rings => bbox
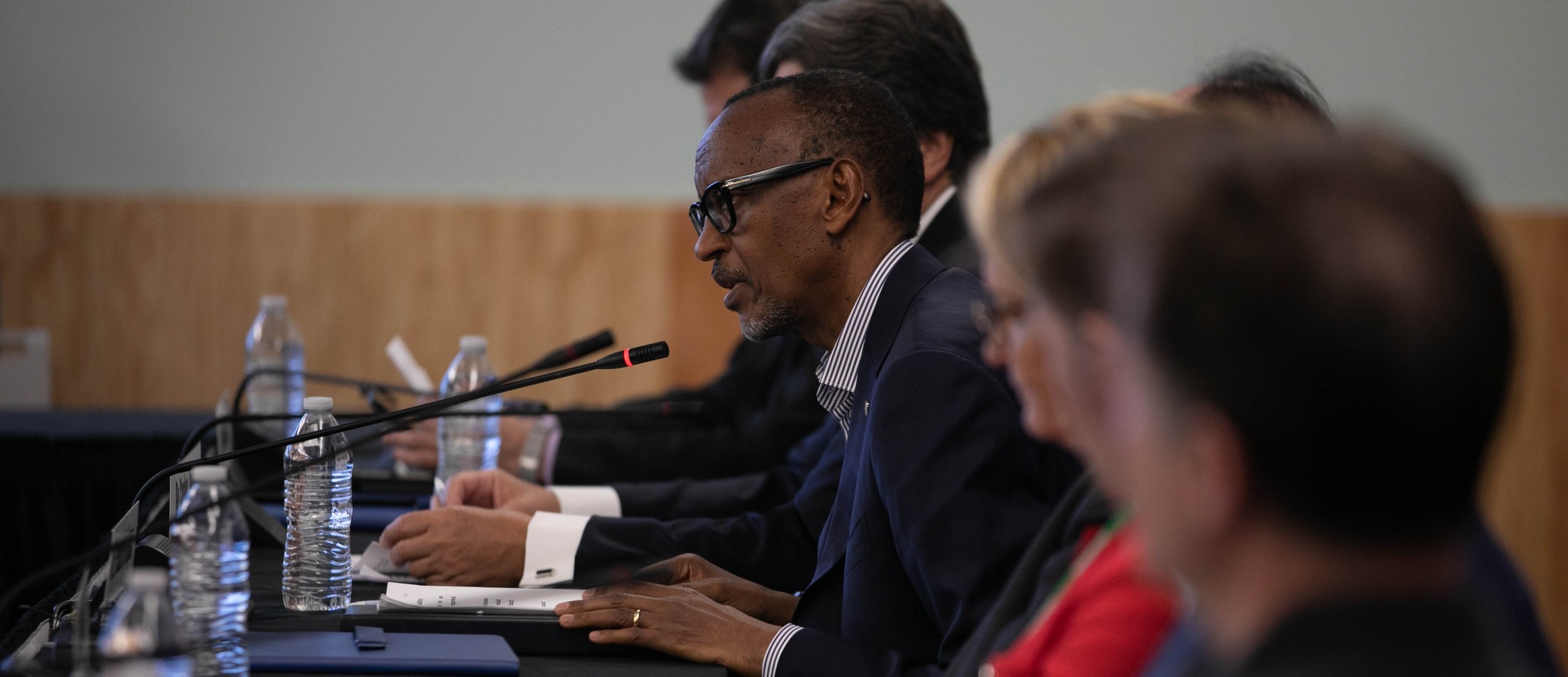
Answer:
[632,608,641,627]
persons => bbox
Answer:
[970,91,1227,677]
[1107,123,1520,677]
[556,64,1039,677]
[367,0,1346,677]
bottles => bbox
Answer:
[98,568,193,677]
[433,336,500,505]
[242,295,304,442]
[281,397,353,611]
[169,466,249,677]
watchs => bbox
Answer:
[516,424,554,487]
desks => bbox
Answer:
[0,405,734,677]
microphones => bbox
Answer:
[132,329,670,531]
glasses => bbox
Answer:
[972,291,1039,349]
[688,158,871,236]
